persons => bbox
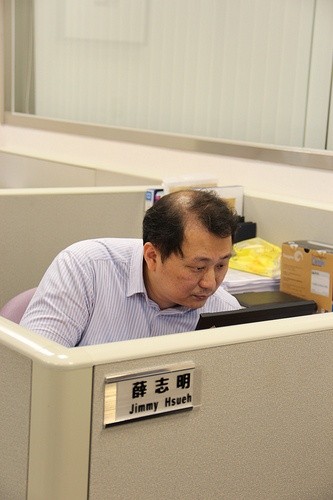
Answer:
[15,191,244,349]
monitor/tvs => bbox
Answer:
[193,301,319,331]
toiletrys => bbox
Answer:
[154,176,177,207]
